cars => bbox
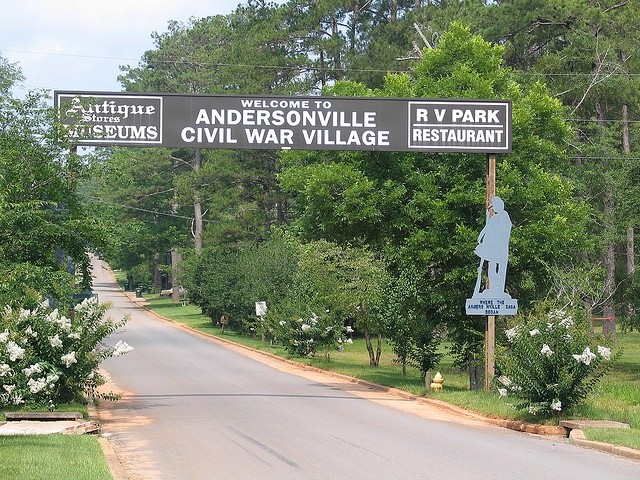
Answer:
[161,286,188,298]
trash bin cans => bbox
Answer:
[136,288,143,297]
[124,282,130,290]
